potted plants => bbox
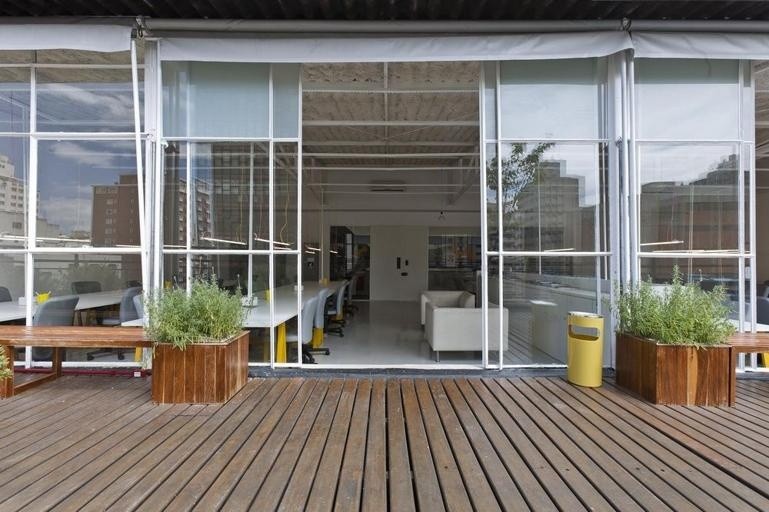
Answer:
[604,264,733,406]
[142,273,253,406]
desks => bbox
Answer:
[233,280,342,361]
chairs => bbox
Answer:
[0,279,140,364]
[418,285,508,361]
[288,274,361,365]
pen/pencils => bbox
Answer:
[35,291,52,295]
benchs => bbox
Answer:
[729,332,769,404]
[1,325,159,397]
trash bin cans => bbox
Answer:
[567,310,604,387]
